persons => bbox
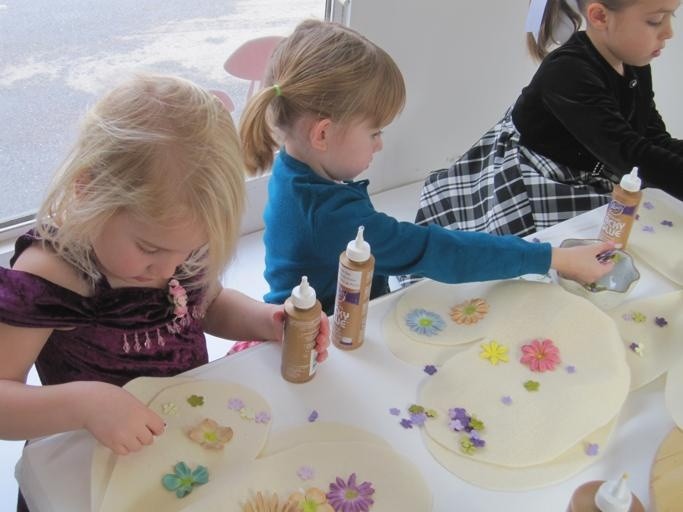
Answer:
[231,18,616,300]
[2,72,334,458]
[401,1,680,236]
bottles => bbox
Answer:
[279,276,322,384]
[598,165,641,250]
[331,226,375,351]
[564,470,646,512]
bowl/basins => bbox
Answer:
[554,236,639,309]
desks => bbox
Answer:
[18,187,680,510]
